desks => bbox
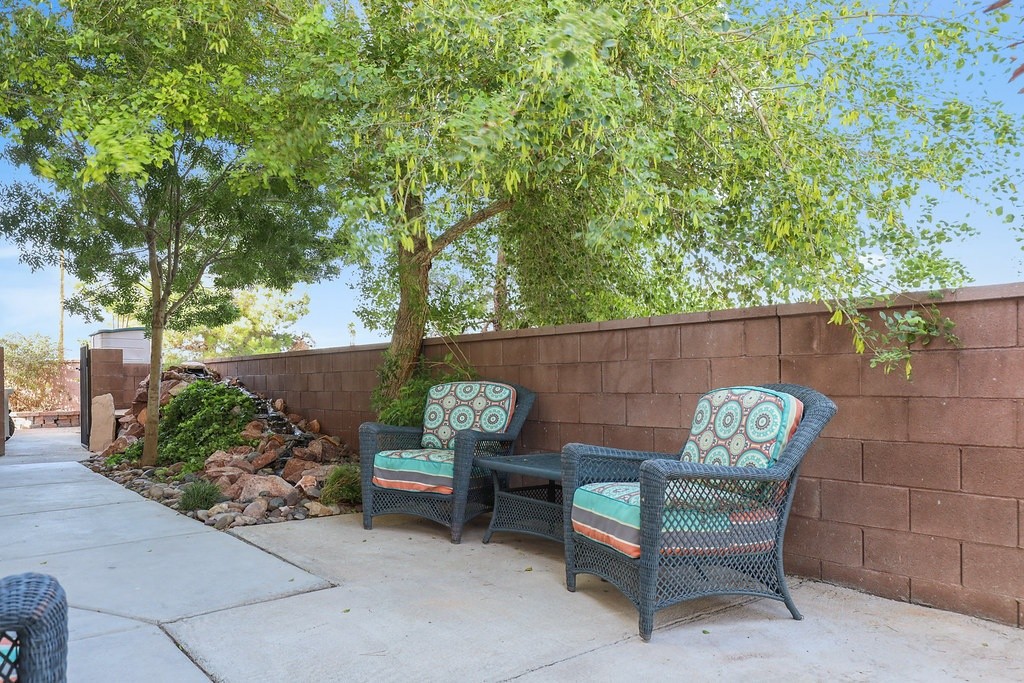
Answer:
[478,453,654,550]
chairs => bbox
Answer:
[563,382,838,641]
[359,379,538,543]
